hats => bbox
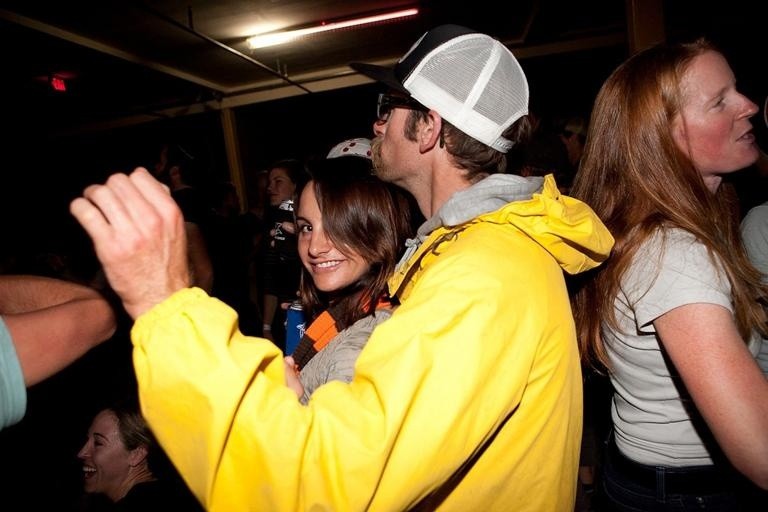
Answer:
[327,137,373,159]
[351,25,529,154]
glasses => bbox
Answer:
[376,93,424,120]
